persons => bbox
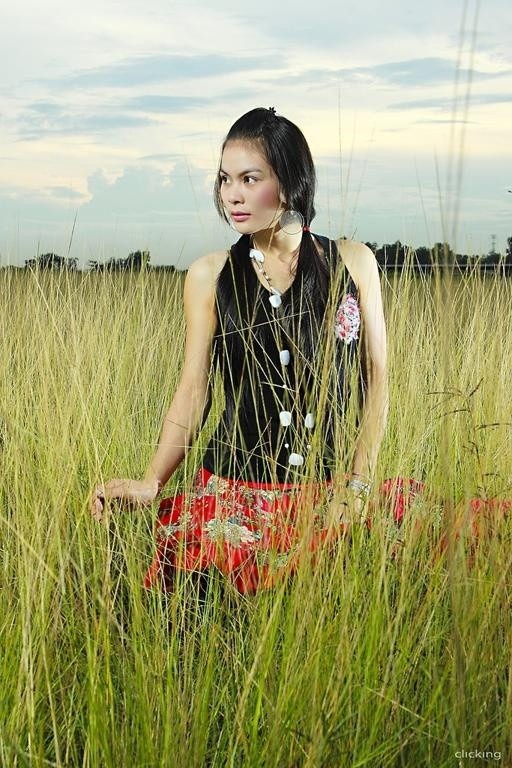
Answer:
[86,103,393,616]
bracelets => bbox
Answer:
[352,471,372,480]
[346,481,371,497]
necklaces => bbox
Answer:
[249,233,319,471]
